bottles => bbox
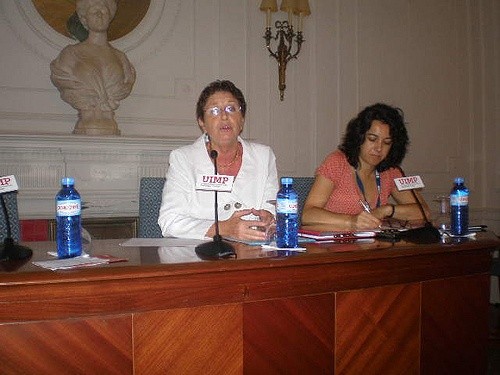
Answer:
[276,177,299,255]
[449,177,469,242]
[55,177,83,259]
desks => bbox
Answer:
[1,235,500,374]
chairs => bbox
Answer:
[138,177,166,237]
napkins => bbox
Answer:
[440,230,476,237]
[261,241,307,252]
[47,250,90,258]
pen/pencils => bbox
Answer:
[359,200,382,228]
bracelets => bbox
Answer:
[386,203,395,218]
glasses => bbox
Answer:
[204,105,241,119]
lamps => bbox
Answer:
[259,0,311,102]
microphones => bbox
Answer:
[194,150,236,257]
[389,162,440,246]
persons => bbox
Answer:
[157,81,280,242]
[301,103,431,230]
[49,0,139,136]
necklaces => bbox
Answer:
[208,139,240,167]
